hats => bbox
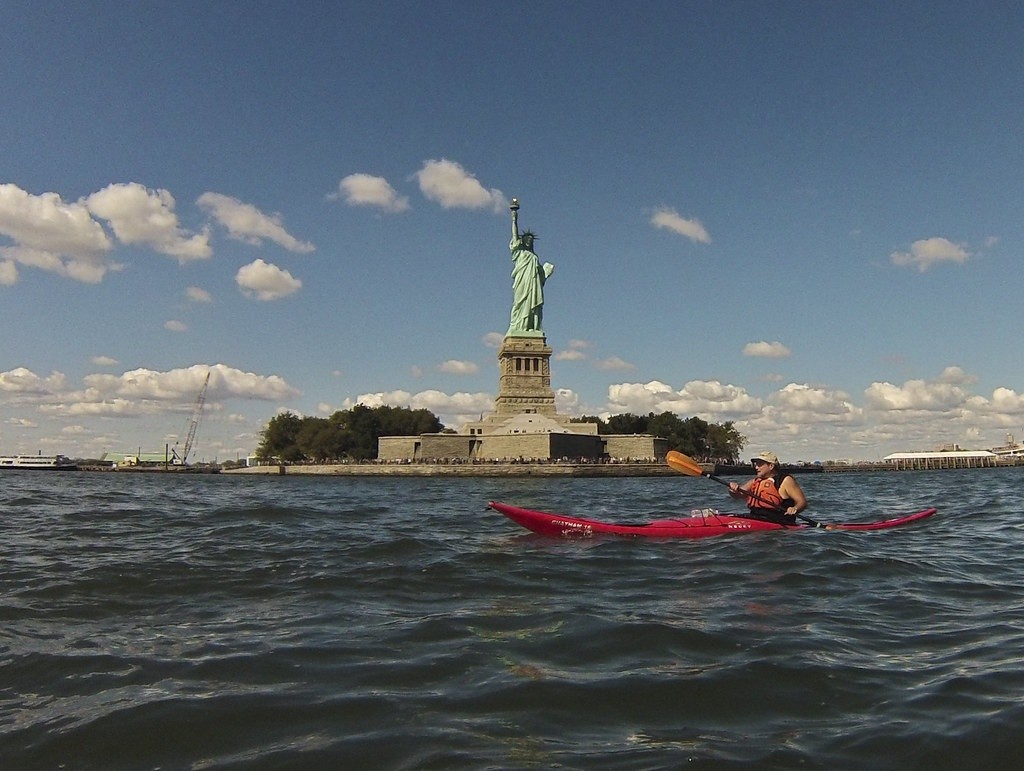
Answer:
[751,452,780,470]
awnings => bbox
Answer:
[883,451,997,461]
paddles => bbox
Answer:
[665,449,832,531]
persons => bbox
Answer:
[506,199,556,336]
[727,451,807,525]
[256,457,739,466]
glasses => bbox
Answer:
[754,460,767,466]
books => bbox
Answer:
[543,262,555,278]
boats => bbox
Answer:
[1,452,80,469]
[485,498,937,536]
